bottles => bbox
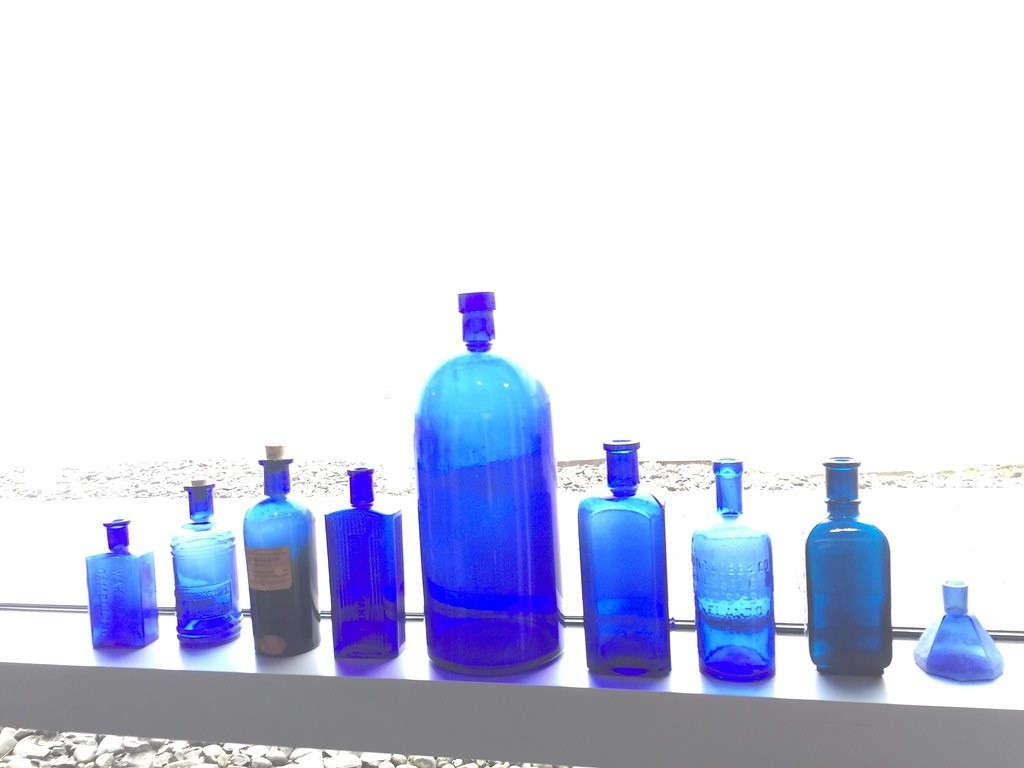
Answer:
[169,479,243,650]
[691,457,775,683]
[243,444,321,658]
[913,579,1004,682]
[324,467,407,659]
[577,440,672,679]
[415,291,565,677]
[85,518,160,649]
[805,454,893,677]
